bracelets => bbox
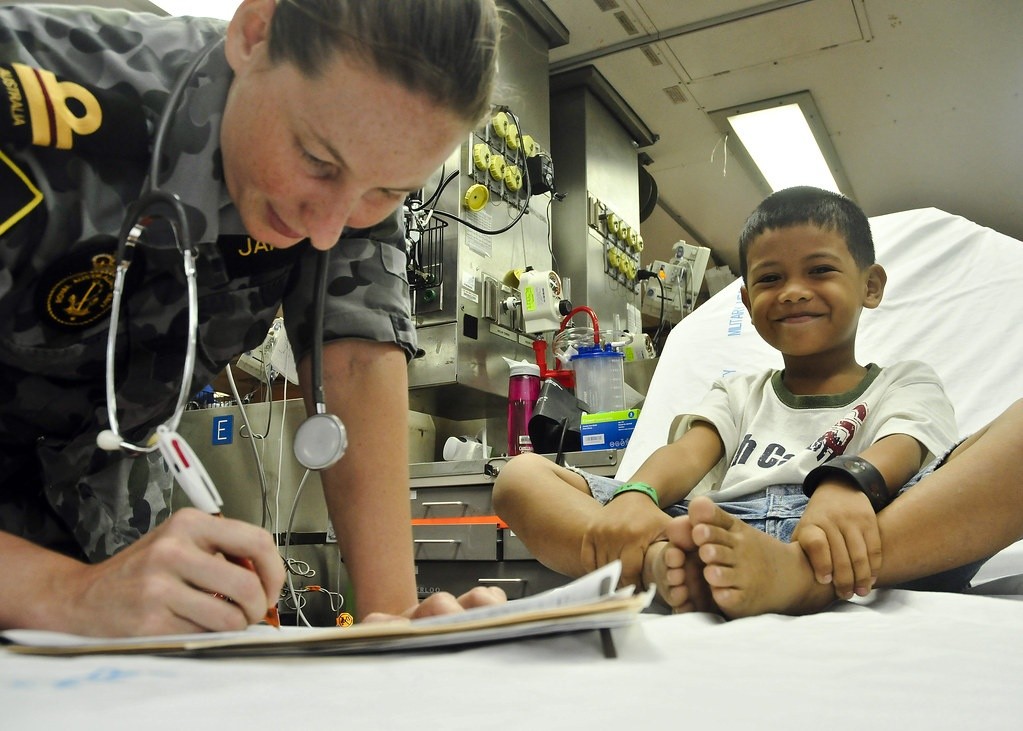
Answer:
[803,455,888,513]
[604,482,659,507]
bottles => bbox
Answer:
[504,357,541,456]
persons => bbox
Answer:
[0,0,505,636]
[492,185,1023,620]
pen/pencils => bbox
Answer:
[158,432,280,631]
